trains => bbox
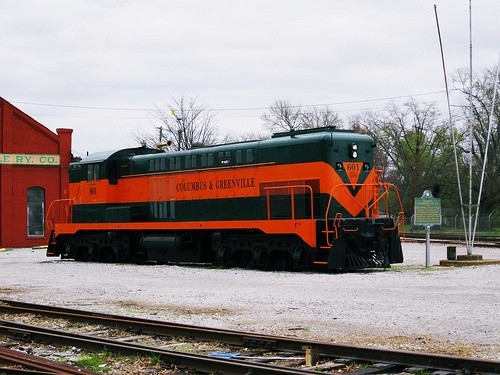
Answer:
[41,122,405,274]
